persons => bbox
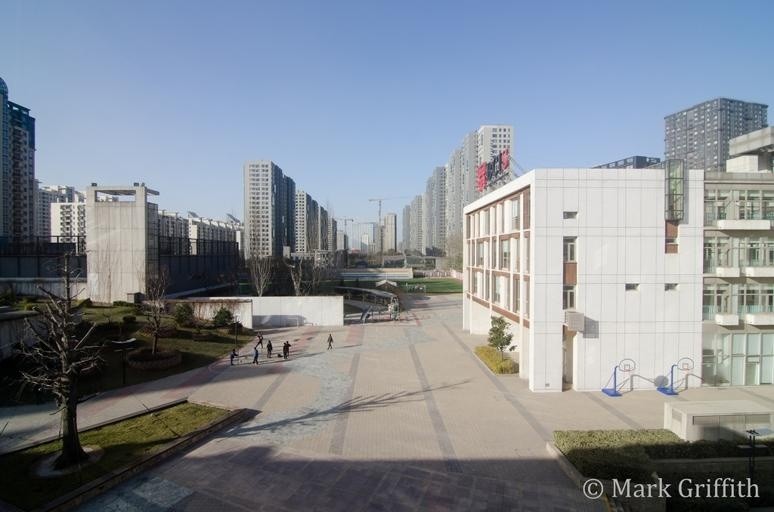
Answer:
[283,342,287,359]
[255,331,263,347]
[266,341,273,358]
[326,333,333,350]
[252,347,258,366]
[285,340,291,356]
[230,347,238,366]
